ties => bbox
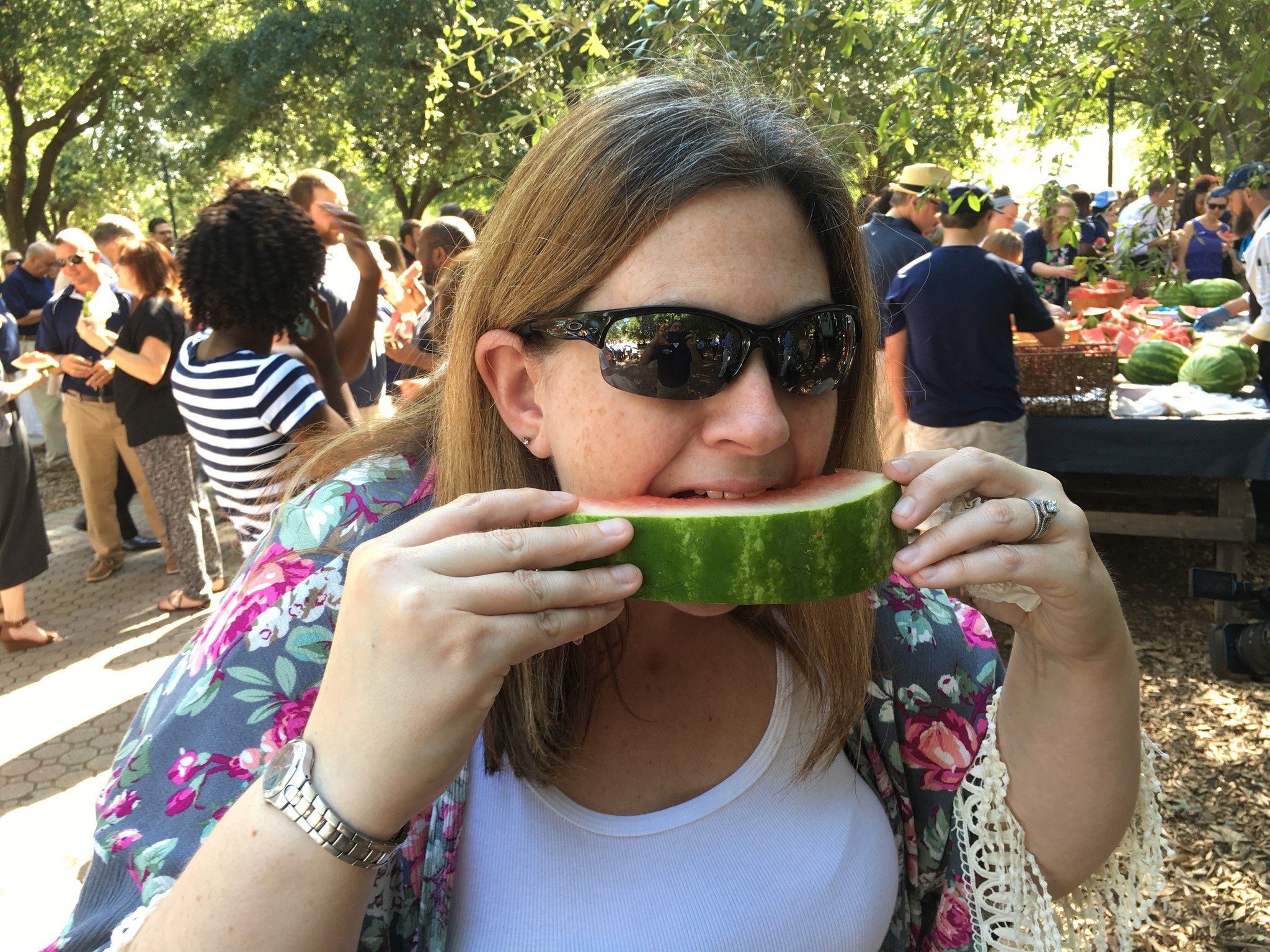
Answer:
[1157,214,1166,275]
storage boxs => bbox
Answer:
[1014,343,1120,417]
[1068,275,1156,313]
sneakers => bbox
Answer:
[167,557,180,574]
[86,546,125,582]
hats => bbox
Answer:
[889,164,951,199]
[1194,174,1221,192]
[1089,190,1118,208]
[1211,161,1270,198]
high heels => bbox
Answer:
[0,611,58,653]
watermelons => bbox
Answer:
[542,469,910,602]
[83,291,94,317]
[1066,278,1259,394]
[1093,237,1107,251]
[1216,230,1240,241]
[1163,228,1186,235]
[11,351,59,372]
[397,260,422,285]
[383,311,417,339]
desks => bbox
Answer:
[1020,378,1270,624]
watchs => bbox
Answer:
[262,737,411,869]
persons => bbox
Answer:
[0,162,1270,653]
[40,75,1169,950]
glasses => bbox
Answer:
[42,260,56,267]
[3,257,24,266]
[117,253,130,266]
[154,229,171,235]
[514,305,859,402]
[1206,202,1227,210]
[54,251,96,268]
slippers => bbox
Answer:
[212,579,229,594]
[158,589,211,611]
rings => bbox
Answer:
[1021,497,1060,541]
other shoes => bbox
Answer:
[72,509,87,532]
[123,535,162,552]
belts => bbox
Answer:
[63,390,115,404]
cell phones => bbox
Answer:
[667,331,691,343]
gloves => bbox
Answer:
[1194,306,1231,332]
